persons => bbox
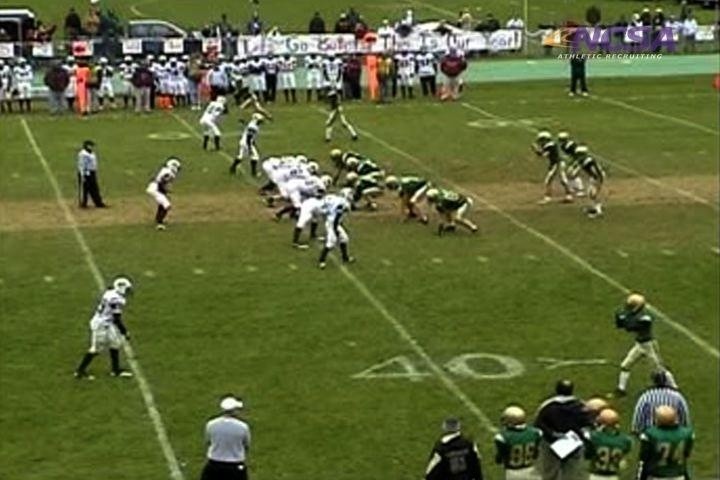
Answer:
[1,0,720,265]
[423,365,696,479]
[605,292,680,400]
[73,276,133,381]
[199,395,251,480]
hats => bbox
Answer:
[219,397,243,409]
[440,414,458,435]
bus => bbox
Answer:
[0,8,35,69]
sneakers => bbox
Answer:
[155,217,166,230]
[254,187,356,268]
[536,188,602,217]
[231,169,259,177]
[202,143,220,150]
[75,368,93,380]
[110,368,130,379]
[403,213,480,235]
[366,201,376,209]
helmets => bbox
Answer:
[253,113,264,122]
[599,409,619,424]
[535,130,587,155]
[585,395,607,409]
[18,53,261,66]
[164,158,180,168]
[425,189,439,200]
[296,149,359,213]
[654,406,676,428]
[497,407,526,429]
[626,293,644,314]
[556,378,573,395]
[215,95,227,104]
[112,276,131,294]
[386,175,398,192]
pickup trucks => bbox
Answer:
[80,17,190,59]
